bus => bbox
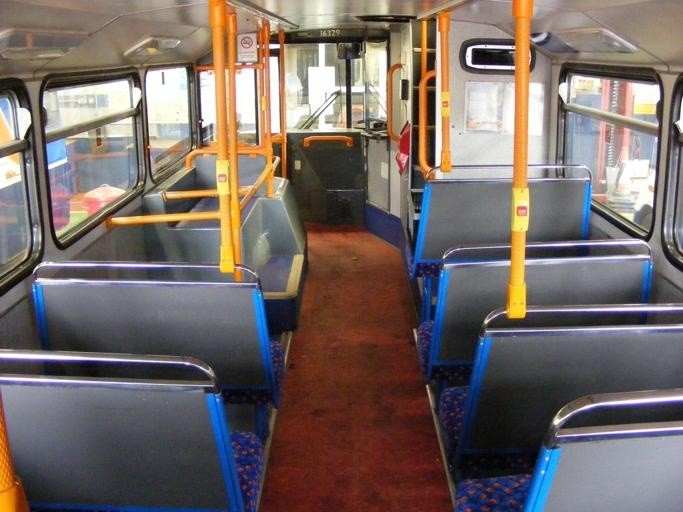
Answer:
[0,91,75,258]
[629,141,683,252]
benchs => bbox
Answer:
[398,164,592,323]
[414,238,654,416]
[30,260,286,416]
[1,335,265,506]
[436,301,683,484]
[451,383,683,509]
